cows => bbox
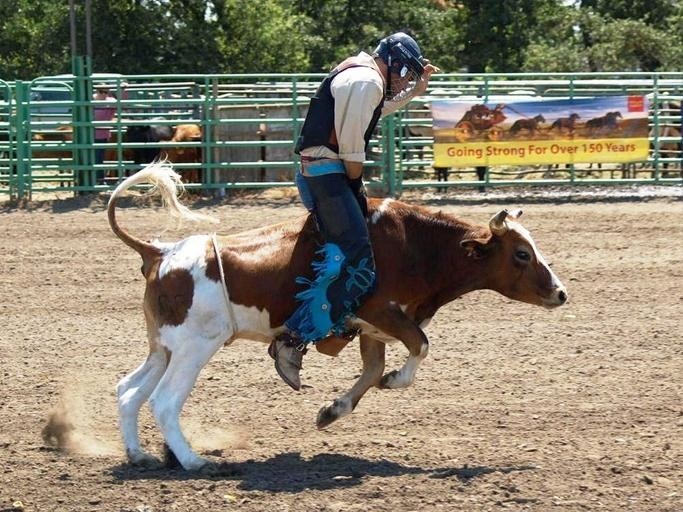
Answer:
[393,96,487,194]
[108,152,569,473]
[1,117,203,193]
[649,103,683,179]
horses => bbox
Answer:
[584,112,622,135]
[509,114,545,137]
[546,114,581,134]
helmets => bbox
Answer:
[373,32,426,103]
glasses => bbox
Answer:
[99,90,108,95]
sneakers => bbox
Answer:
[268,329,303,391]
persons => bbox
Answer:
[91,82,128,184]
[264,31,440,390]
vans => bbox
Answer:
[26,72,127,133]
